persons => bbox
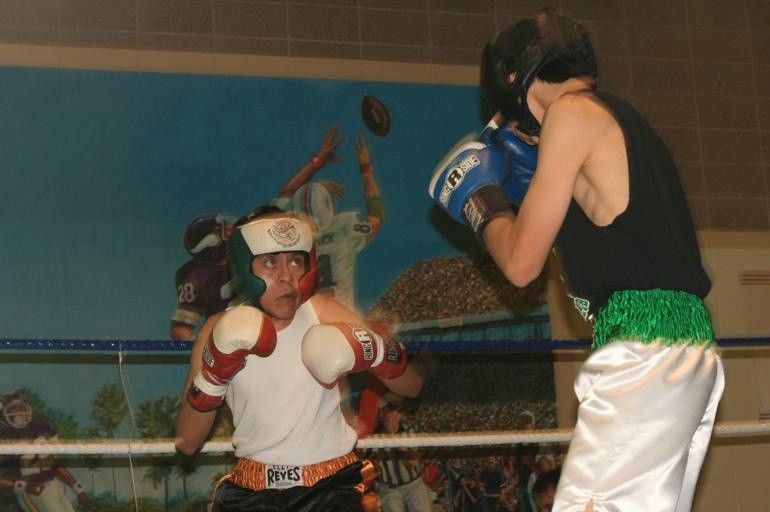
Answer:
[174,204,423,512]
[367,255,550,327]
[171,179,345,341]
[0,393,88,512]
[272,125,386,427]
[425,411,561,511]
[429,9,725,512]
[366,400,433,510]
[368,351,559,435]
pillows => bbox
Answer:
[186,214,230,263]
[479,17,598,135]
[226,217,317,310]
[291,182,333,231]
[1,392,33,430]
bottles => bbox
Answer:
[378,392,404,408]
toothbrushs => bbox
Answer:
[361,95,389,137]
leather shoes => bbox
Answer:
[428,126,517,253]
[492,123,538,207]
[186,304,277,412]
[301,322,408,390]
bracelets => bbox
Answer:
[311,153,324,169]
[359,163,373,175]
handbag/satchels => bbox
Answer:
[311,153,324,169]
[359,163,373,175]
